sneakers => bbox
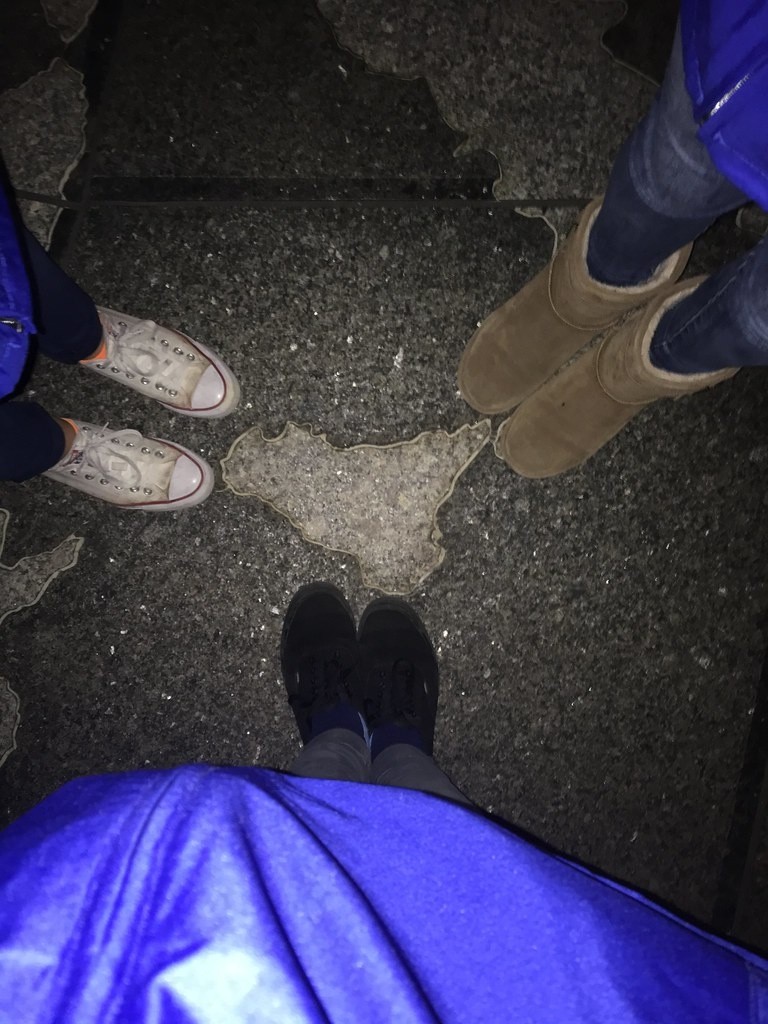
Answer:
[39,418,215,511]
[279,582,440,752]
[79,304,241,418]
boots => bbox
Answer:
[458,191,741,479]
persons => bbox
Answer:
[0,582,768,1024]
[0,185,240,511]
[460,0,768,479]
